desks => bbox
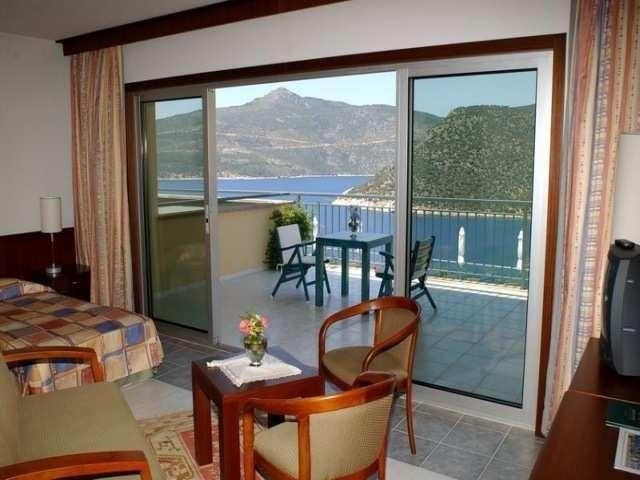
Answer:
[191,345,326,479]
[528,336,639,479]
[315,233,393,307]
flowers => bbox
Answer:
[239,311,269,357]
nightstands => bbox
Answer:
[28,261,90,299]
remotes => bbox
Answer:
[615,238,635,250]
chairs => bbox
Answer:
[270,223,330,301]
[244,292,421,480]
[0,346,164,480]
[373,235,436,308]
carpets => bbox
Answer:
[140,411,222,480]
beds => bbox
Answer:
[1,276,157,396]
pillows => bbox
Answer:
[1,278,48,302]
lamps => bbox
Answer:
[40,196,63,275]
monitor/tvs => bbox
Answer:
[600,243,640,377]
